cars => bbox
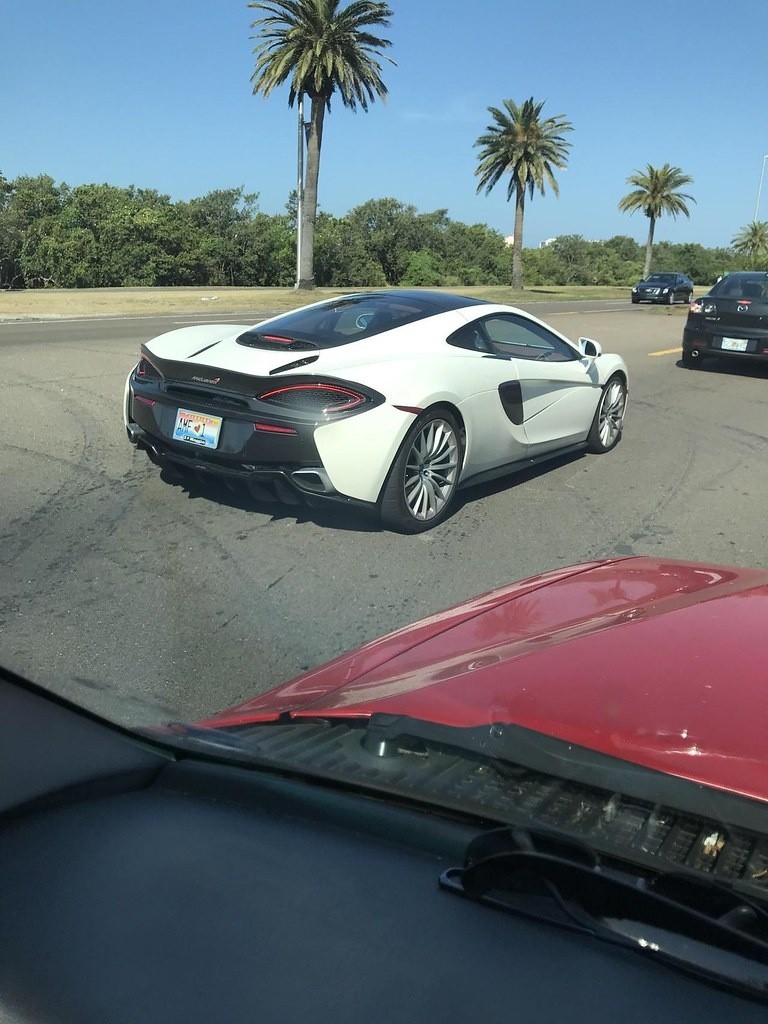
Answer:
[631,272,694,305]
[682,270,768,368]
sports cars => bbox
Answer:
[123,287,631,537]
